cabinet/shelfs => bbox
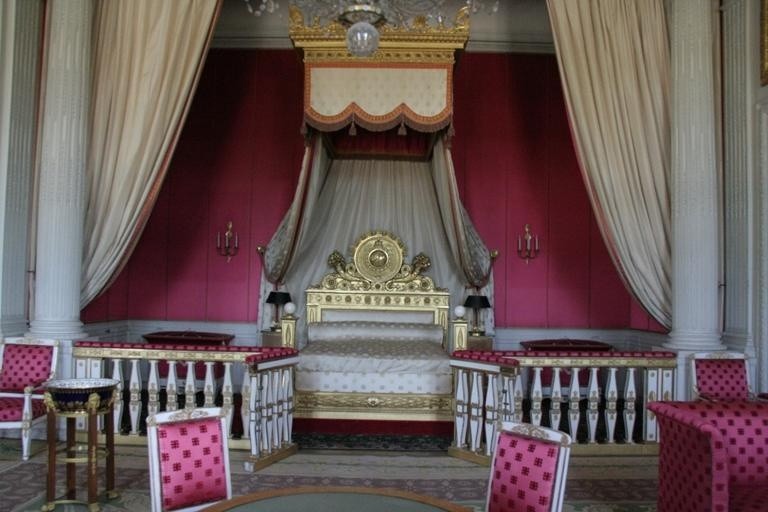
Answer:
[43,388,123,512]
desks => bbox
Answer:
[192,486,475,511]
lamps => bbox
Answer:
[266,291,292,333]
[463,295,493,337]
[244,0,500,56]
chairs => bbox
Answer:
[145,403,233,511]
[487,420,573,511]
[687,351,756,398]
[1,336,60,461]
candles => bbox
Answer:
[516,223,539,266]
[217,221,240,264]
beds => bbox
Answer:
[293,230,455,420]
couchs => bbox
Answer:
[647,398,768,512]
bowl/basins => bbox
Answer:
[45,378,120,414]
